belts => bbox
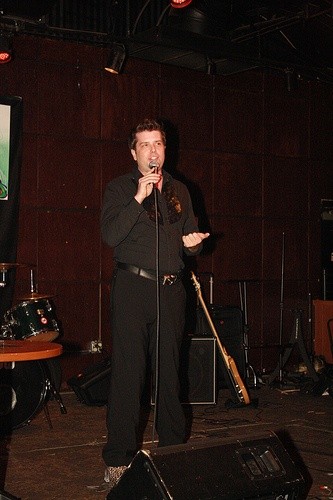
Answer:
[117,262,182,286]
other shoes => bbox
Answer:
[106,465,128,488]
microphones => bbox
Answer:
[149,160,160,190]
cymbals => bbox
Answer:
[16,292,56,300]
[0,262,37,270]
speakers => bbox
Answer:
[105,429,305,500]
[70,361,111,406]
[149,306,246,406]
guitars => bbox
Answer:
[191,270,248,404]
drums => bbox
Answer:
[3,300,60,341]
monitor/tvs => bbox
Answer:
[0,96,22,211]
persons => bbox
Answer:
[102,117,211,484]
